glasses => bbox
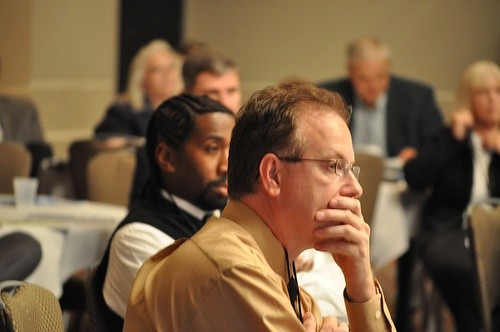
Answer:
[278,156,361,180]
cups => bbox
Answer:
[12,177,38,209]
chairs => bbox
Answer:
[0,140,33,194]
[462,194,500,332]
[70,139,138,207]
[0,232,66,332]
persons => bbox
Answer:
[125,81,396,332]
[403,61,500,332]
[66,92,236,332]
[311,35,446,166]
[0,94,54,195]
[182,49,244,115]
[92,40,187,152]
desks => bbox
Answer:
[0,191,127,283]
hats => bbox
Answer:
[0,280,67,332]
